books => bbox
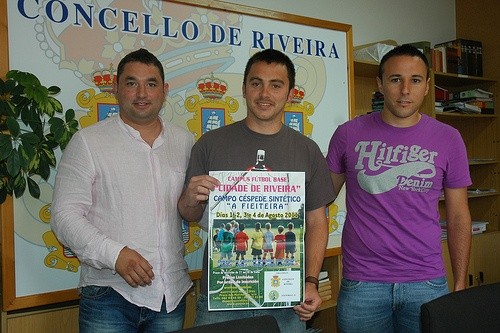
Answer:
[372,40,497,239]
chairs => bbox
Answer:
[419,282,500,333]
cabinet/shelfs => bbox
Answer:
[0,248,348,333]
[354,40,500,293]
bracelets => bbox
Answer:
[305,276,319,290]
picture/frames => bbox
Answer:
[0,0,355,312]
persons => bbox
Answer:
[178,49,336,333]
[325,44,472,333]
[50,48,208,333]
[214,220,297,266]
